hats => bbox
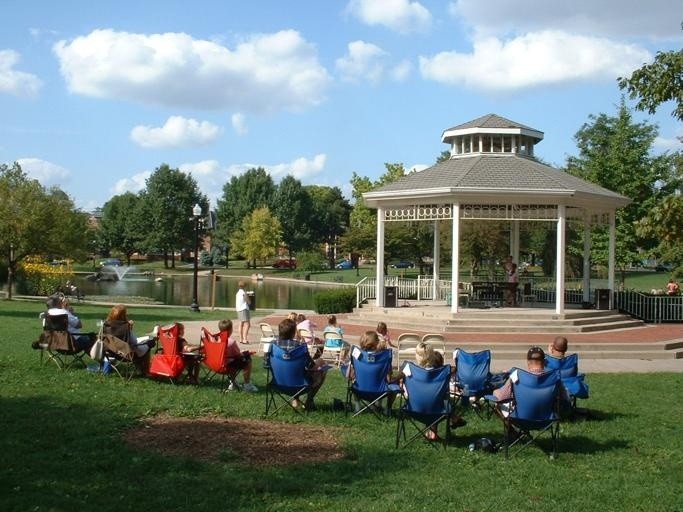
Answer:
[527,348,545,360]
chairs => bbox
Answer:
[387,362,454,452]
[39,313,244,393]
[341,345,391,422]
[258,324,446,371]
[453,347,510,422]
[483,368,562,460]
[520,279,537,308]
[263,342,332,419]
[458,283,469,307]
[545,356,589,409]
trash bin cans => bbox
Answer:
[305,271,311,281]
[595,289,611,310]
[384,286,398,308]
[246,291,256,310]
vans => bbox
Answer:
[272,258,297,269]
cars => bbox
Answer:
[485,259,544,268]
[335,259,355,269]
[391,261,414,269]
[49,256,65,265]
[320,262,331,270]
[99,258,123,268]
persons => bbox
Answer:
[178,323,202,386]
[666,278,678,296]
[108,305,160,360]
[401,343,485,441]
[323,315,349,366]
[494,346,569,432]
[270,314,326,411]
[218,319,259,392]
[484,256,519,307]
[347,321,405,414]
[548,336,568,359]
[46,296,116,366]
[235,279,252,344]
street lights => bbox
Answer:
[189,203,203,313]
[93,240,96,270]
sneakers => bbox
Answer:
[451,419,467,429]
[242,383,258,391]
[228,383,242,391]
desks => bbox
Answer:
[471,282,519,307]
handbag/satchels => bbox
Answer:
[90,339,103,361]
